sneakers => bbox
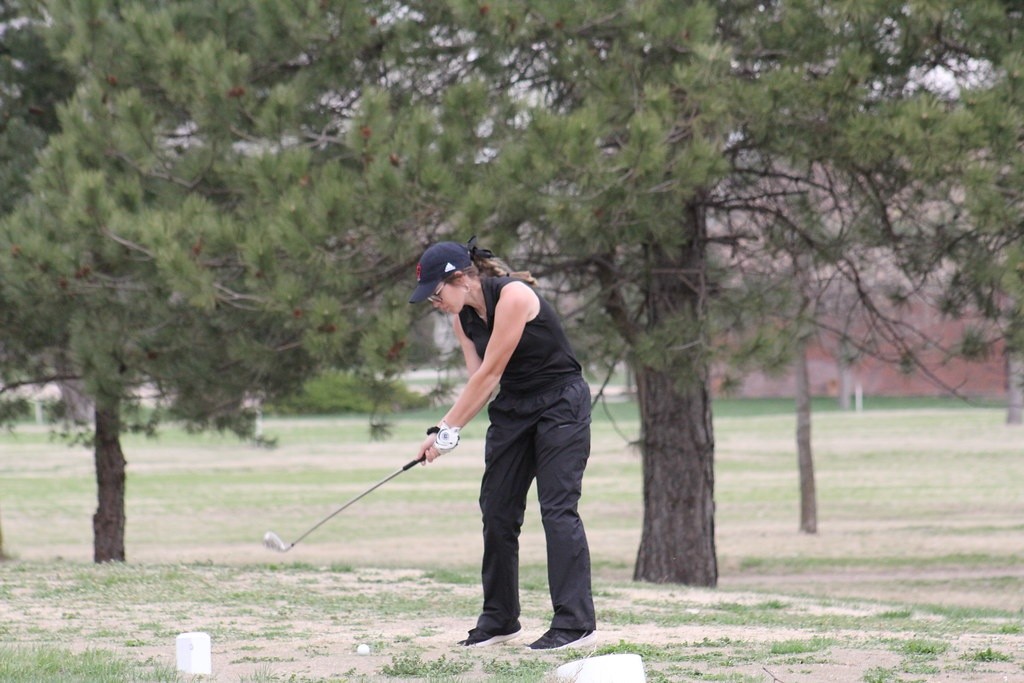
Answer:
[456,621,522,648]
[526,628,597,652]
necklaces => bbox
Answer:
[476,302,488,318]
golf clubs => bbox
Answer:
[262,452,427,554]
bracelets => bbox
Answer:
[426,426,441,435]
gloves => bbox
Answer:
[433,438,457,455]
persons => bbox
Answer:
[409,235,601,652]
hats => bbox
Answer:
[410,242,471,304]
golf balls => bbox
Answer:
[357,644,370,656]
